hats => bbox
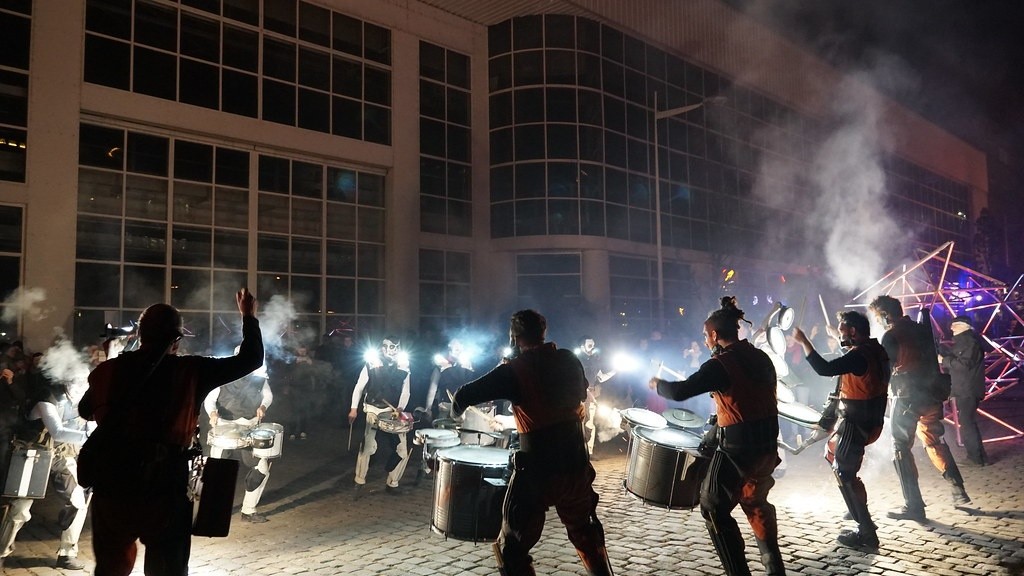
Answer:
[951,315,972,326]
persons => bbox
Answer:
[76,286,264,576]
[415,322,708,479]
[793,310,892,546]
[203,345,274,522]
[276,333,412,494]
[868,293,971,519]
[0,326,121,569]
[451,308,616,576]
[739,312,840,479]
[649,295,786,576]
[936,316,990,466]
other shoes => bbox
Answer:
[352,481,364,498]
[955,455,989,467]
[887,507,926,521]
[289,433,299,441]
[838,529,879,547]
[241,513,266,523]
[590,455,599,461]
[300,432,307,440]
[56,556,85,569]
[951,492,970,504]
[384,484,402,495]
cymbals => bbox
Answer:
[457,428,505,439]
[662,408,705,428]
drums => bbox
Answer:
[620,408,667,432]
[190,456,240,538]
[766,378,795,405]
[376,411,415,433]
[776,399,823,424]
[766,326,787,355]
[249,430,275,449]
[413,428,461,447]
[250,422,283,460]
[430,443,515,543]
[206,423,252,450]
[622,424,711,510]
[434,402,497,446]
[779,305,795,331]
[759,346,789,381]
